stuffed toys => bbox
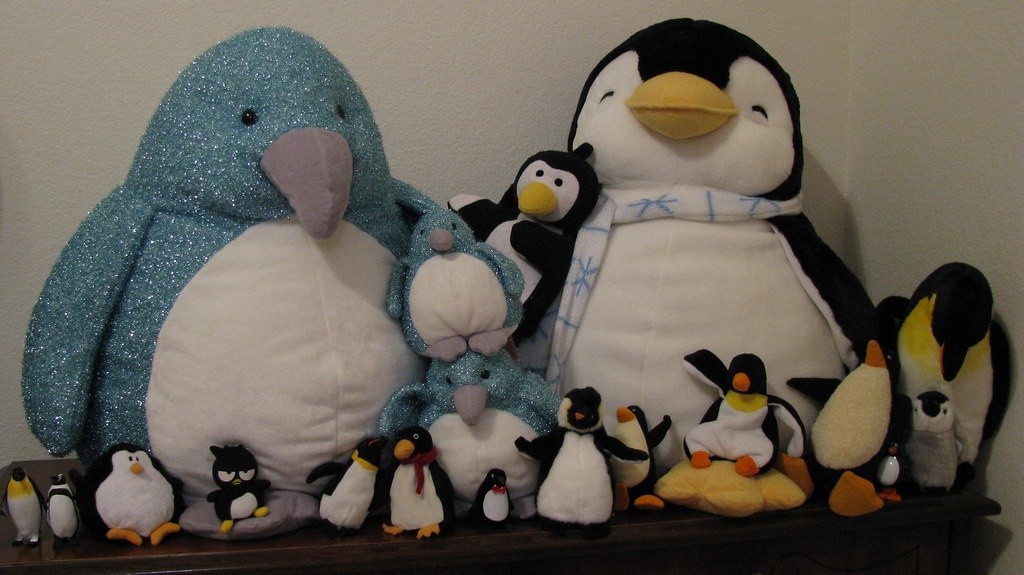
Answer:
[447,140,600,345]
[469,468,515,533]
[681,346,807,477]
[513,386,649,541]
[305,435,390,538]
[205,443,272,534]
[0,466,50,545]
[786,260,1014,517]
[520,14,880,516]
[384,206,526,360]
[381,425,457,542]
[604,404,674,509]
[378,343,566,521]
[45,472,81,550]
[74,442,187,547]
[20,25,455,537]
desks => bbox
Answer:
[0,459,1002,575]
